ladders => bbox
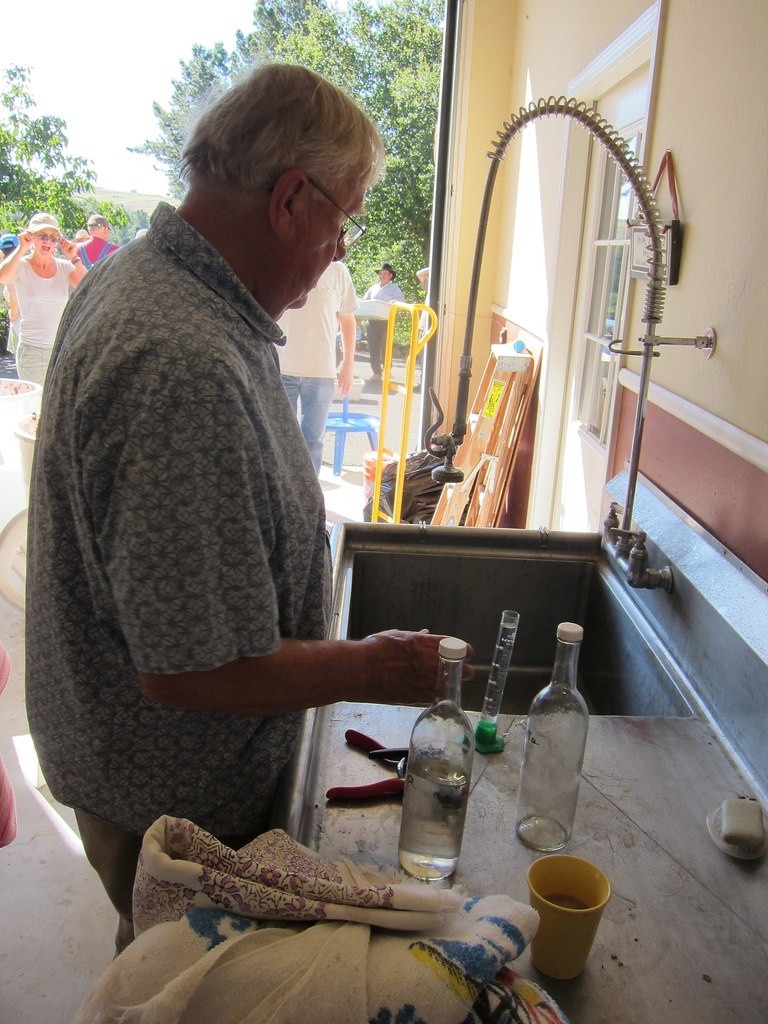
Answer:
[432,344,533,528]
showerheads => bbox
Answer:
[418,381,469,484]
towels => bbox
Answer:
[66,812,571,1024]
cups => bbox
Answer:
[526,854,613,981]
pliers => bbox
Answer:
[326,729,405,801]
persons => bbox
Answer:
[269,262,360,478]
[0,213,122,387]
[411,266,430,392]
[23,67,475,964]
[361,262,404,381]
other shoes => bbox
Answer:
[412,383,422,394]
[369,372,382,381]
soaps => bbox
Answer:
[722,797,764,848]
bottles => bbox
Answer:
[515,622,590,852]
[398,637,477,883]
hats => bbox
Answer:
[70,229,90,243]
[135,228,150,238]
[26,212,61,235]
[374,263,396,281]
[86,214,113,231]
[0,234,19,250]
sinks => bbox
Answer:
[337,521,695,718]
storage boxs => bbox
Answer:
[332,377,365,401]
[350,299,391,321]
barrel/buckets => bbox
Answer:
[362,451,397,501]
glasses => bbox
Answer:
[33,233,58,243]
[266,166,369,250]
